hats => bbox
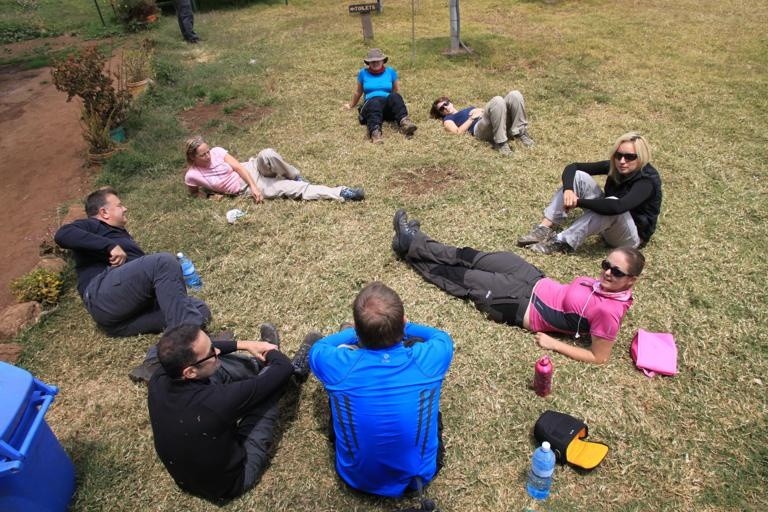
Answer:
[364,48,388,65]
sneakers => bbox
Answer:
[129,362,162,384]
[519,226,558,244]
[260,323,280,350]
[530,237,565,253]
[289,330,323,382]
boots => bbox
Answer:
[371,130,383,143]
[494,141,512,154]
[392,210,418,253]
[515,129,537,146]
[342,187,364,200]
[400,116,417,135]
[396,220,420,257]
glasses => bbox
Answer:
[602,260,634,278]
[438,100,449,111]
[614,151,638,161]
[183,342,218,368]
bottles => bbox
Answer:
[534,357,552,397]
[527,441,556,500]
[176,252,202,289]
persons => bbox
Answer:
[339,49,419,145]
[392,209,646,364]
[184,138,365,205]
[308,281,455,500]
[53,188,213,338]
[428,90,535,157]
[147,323,324,505]
[518,131,663,257]
[175,0,207,45]
